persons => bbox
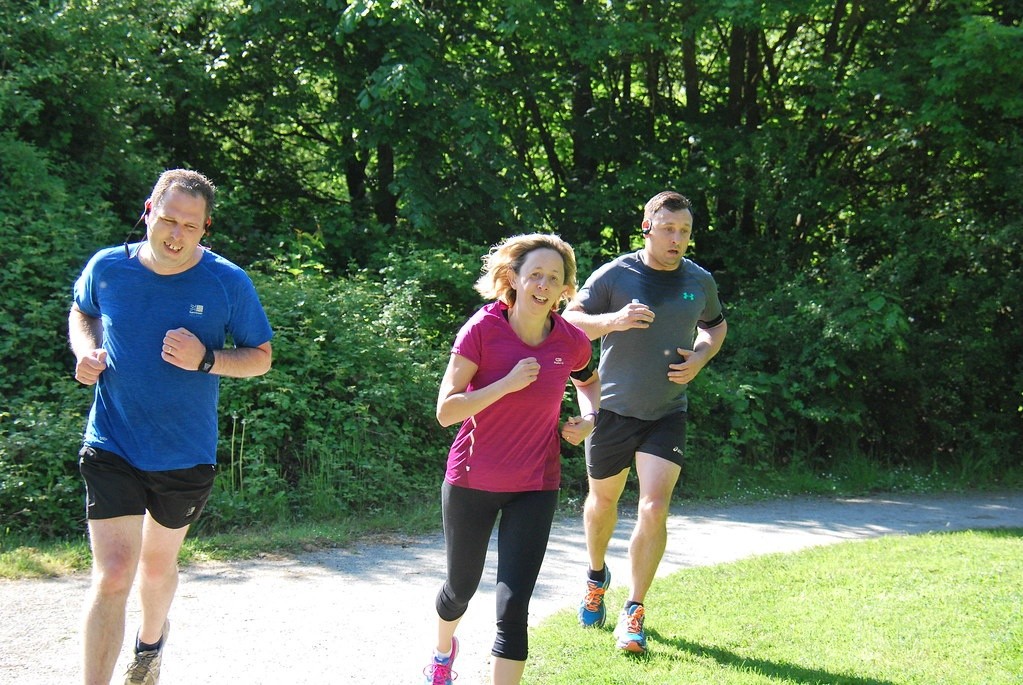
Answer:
[423,233,601,685]
[68,168,276,685]
[561,190,728,654]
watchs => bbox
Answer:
[199,347,215,373]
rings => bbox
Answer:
[566,436,569,440]
[169,348,172,353]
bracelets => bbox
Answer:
[584,409,599,426]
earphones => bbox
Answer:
[643,228,651,233]
[140,207,150,219]
[204,223,210,235]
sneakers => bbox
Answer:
[422,638,459,685]
[123,616,171,685]
[614,603,646,652]
[578,565,611,631]
